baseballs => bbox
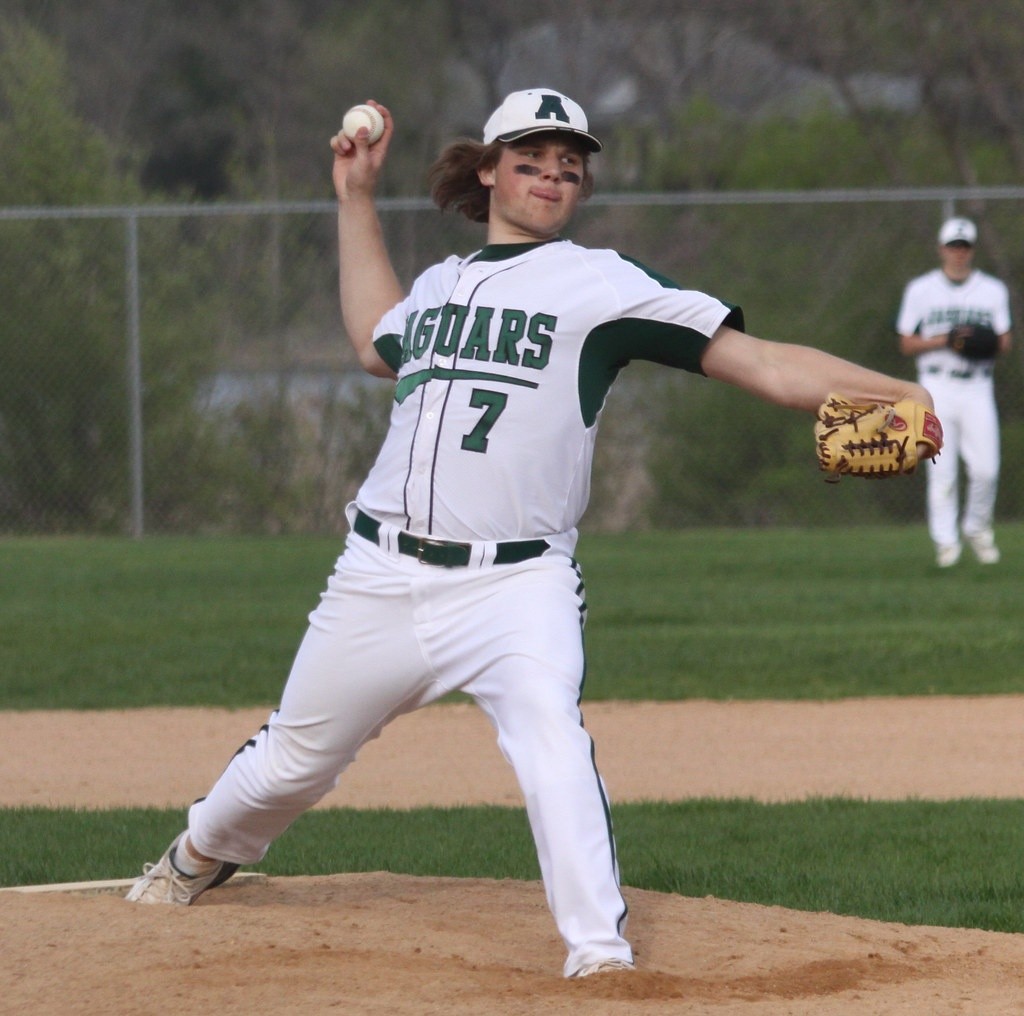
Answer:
[342,102,387,148]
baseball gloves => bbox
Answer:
[947,321,1001,362]
[813,392,943,483]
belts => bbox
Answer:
[354,510,549,567]
[930,367,990,378]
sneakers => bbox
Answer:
[124,828,241,908]
[575,960,635,977]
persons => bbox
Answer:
[895,219,1012,568]
[127,87,942,981]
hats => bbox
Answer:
[938,218,977,247]
[484,88,603,153]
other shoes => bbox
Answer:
[972,542,1001,567]
[936,543,961,567]
